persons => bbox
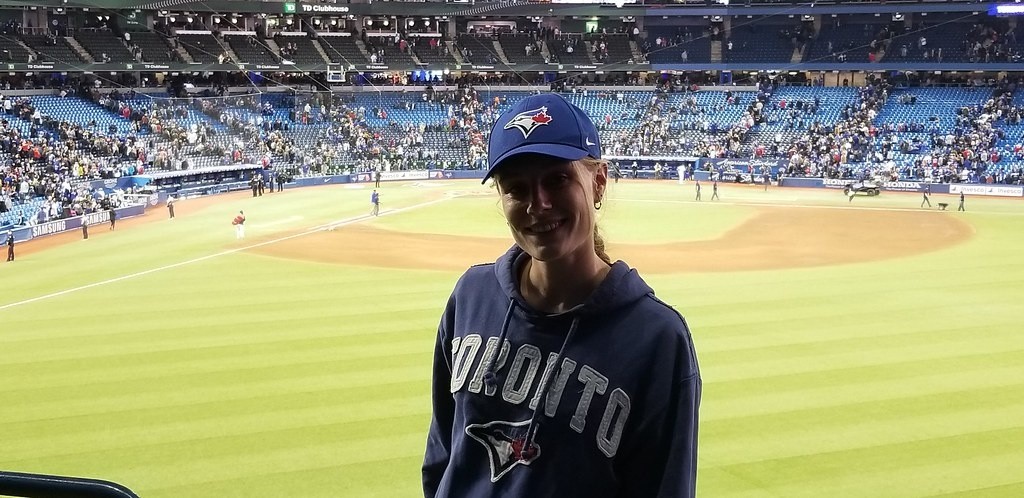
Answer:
[422,94,702,498]
[0,14,1024,261]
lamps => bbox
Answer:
[531,17,544,22]
[710,15,723,22]
[623,15,636,22]
[801,14,814,21]
[892,12,905,21]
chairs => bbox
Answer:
[0,25,1024,230]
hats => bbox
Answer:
[481,94,602,186]
